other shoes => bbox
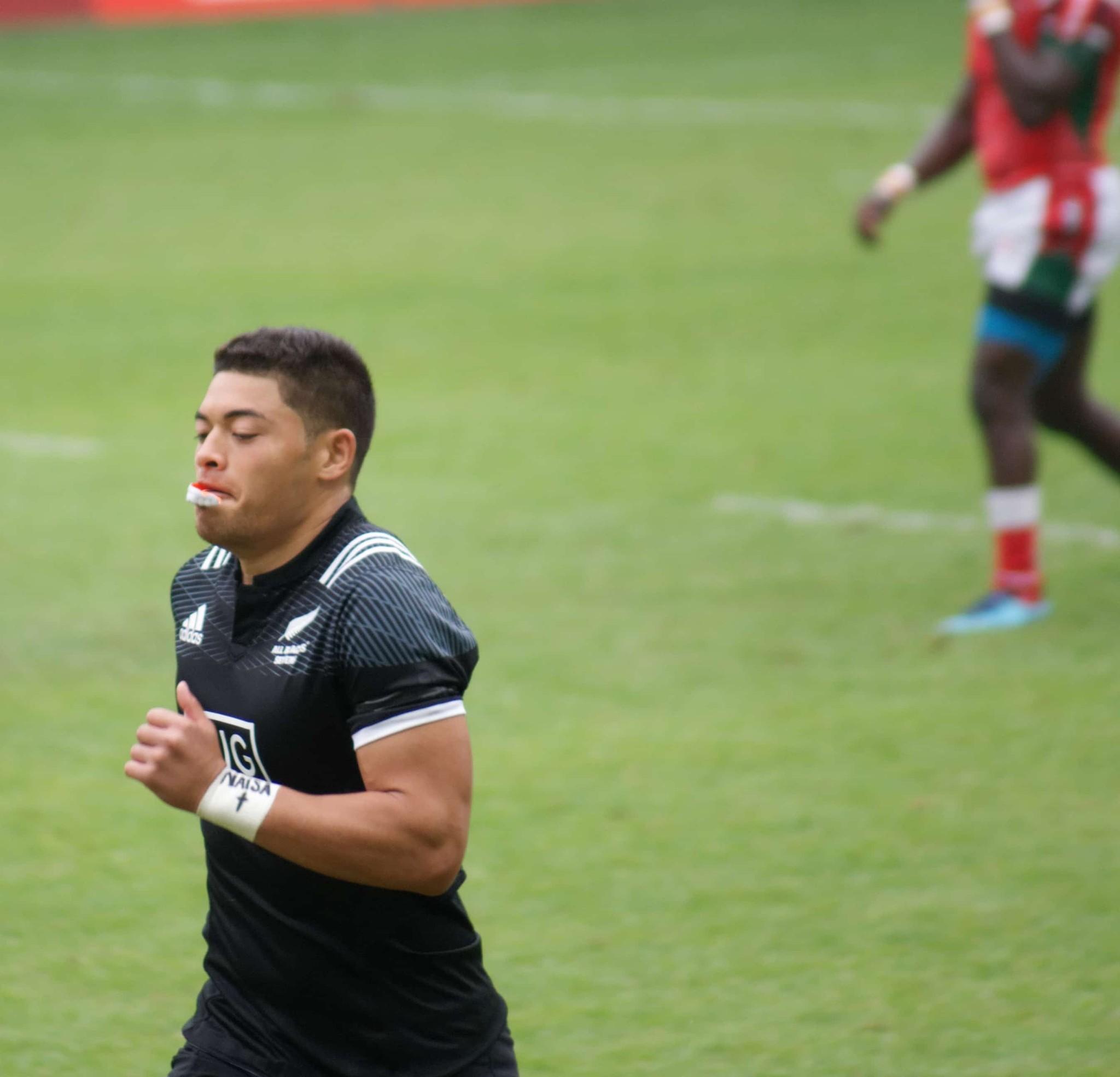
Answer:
[946,591,1047,631]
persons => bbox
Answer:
[124,325,523,1076]
[851,0,1120,637]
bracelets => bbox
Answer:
[979,8,1010,35]
[192,768,282,845]
